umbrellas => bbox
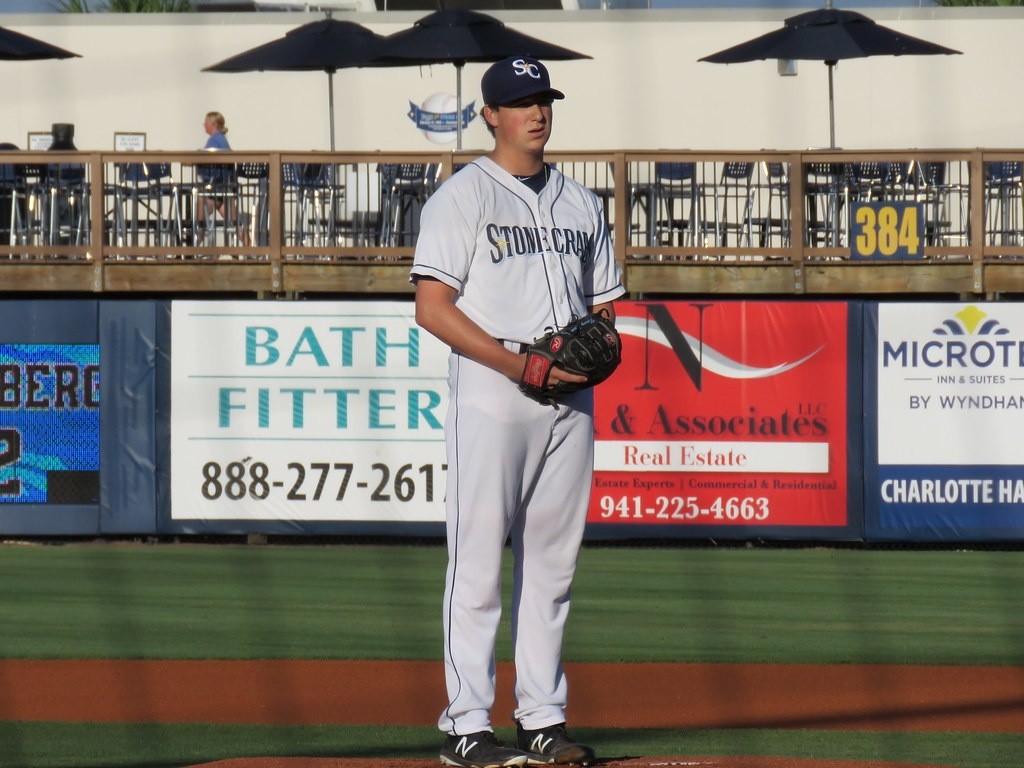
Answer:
[696,10,965,261]
[0,25,84,62]
[200,18,387,246]
[346,6,595,169]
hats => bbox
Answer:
[480,60,565,106]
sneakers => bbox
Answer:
[440,730,528,768]
[513,721,595,766]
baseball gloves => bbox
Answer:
[524,305,623,412]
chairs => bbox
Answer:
[0,139,1024,266]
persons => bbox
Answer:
[182,112,252,259]
[407,55,626,768]
